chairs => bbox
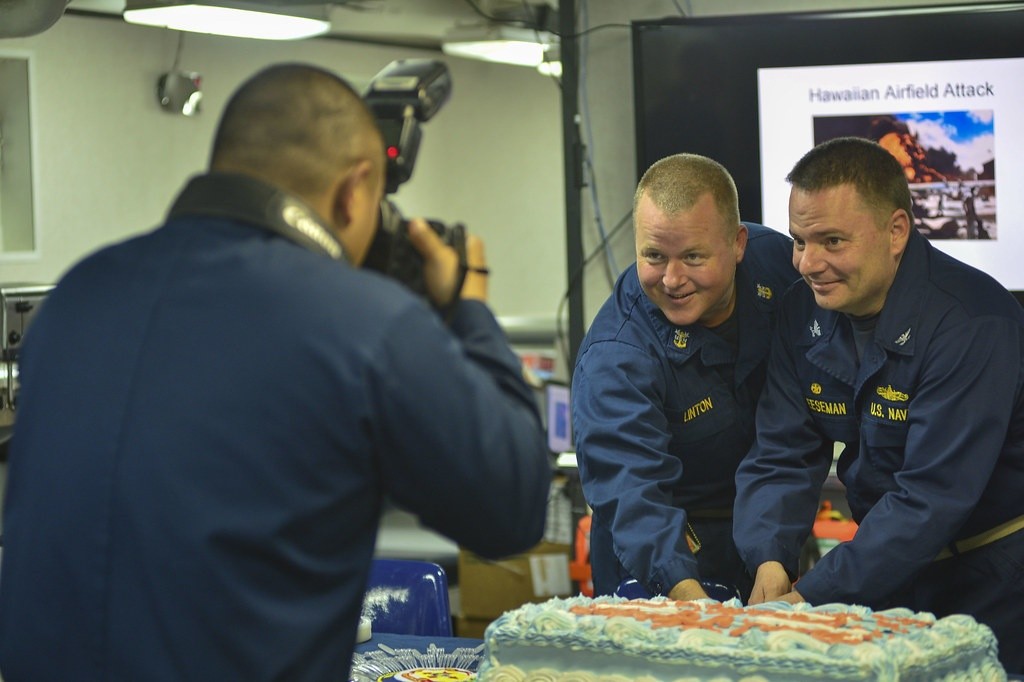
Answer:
[359,555,453,638]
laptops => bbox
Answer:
[543,380,575,457]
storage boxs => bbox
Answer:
[459,540,571,619]
[455,614,491,639]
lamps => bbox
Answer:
[159,31,203,116]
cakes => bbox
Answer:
[474,593,1007,682]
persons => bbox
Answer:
[566,135,1024,681]
[0,59,556,681]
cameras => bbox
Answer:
[361,58,465,297]
